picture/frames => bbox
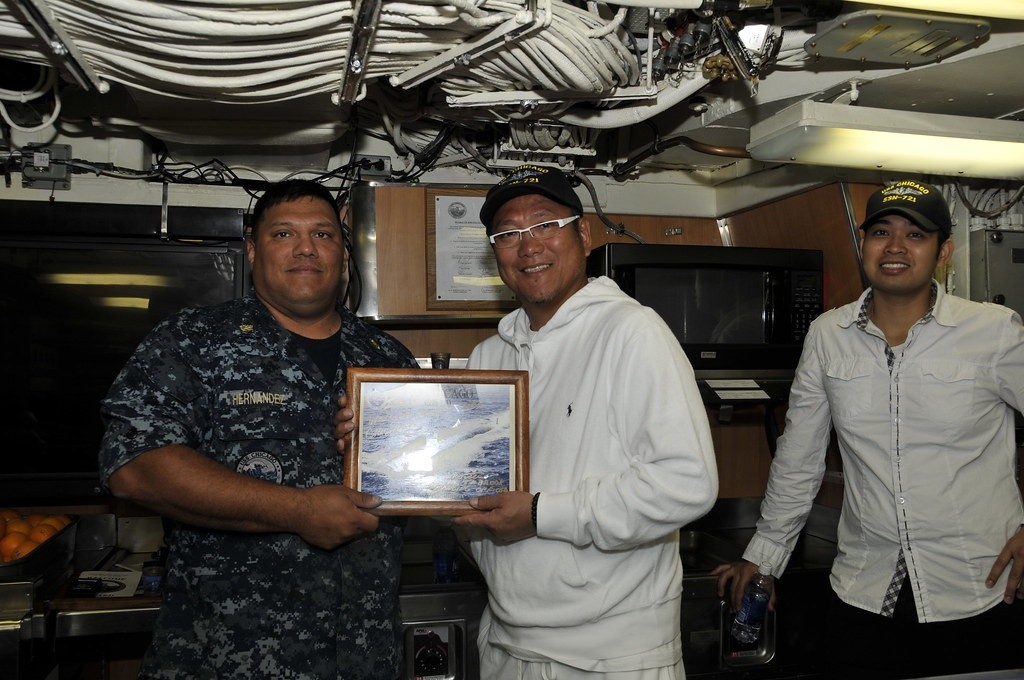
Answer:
[347,369,529,516]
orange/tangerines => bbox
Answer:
[0,509,69,563]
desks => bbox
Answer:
[0,526,840,680]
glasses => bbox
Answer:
[489,214,580,249]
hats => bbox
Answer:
[479,164,583,228]
[859,179,952,239]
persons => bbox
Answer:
[710,180,1024,680]
[333,165,718,680]
[98,177,420,680]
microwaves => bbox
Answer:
[585,243,824,379]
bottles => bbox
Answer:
[731,561,772,643]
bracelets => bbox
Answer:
[531,492,540,528]
